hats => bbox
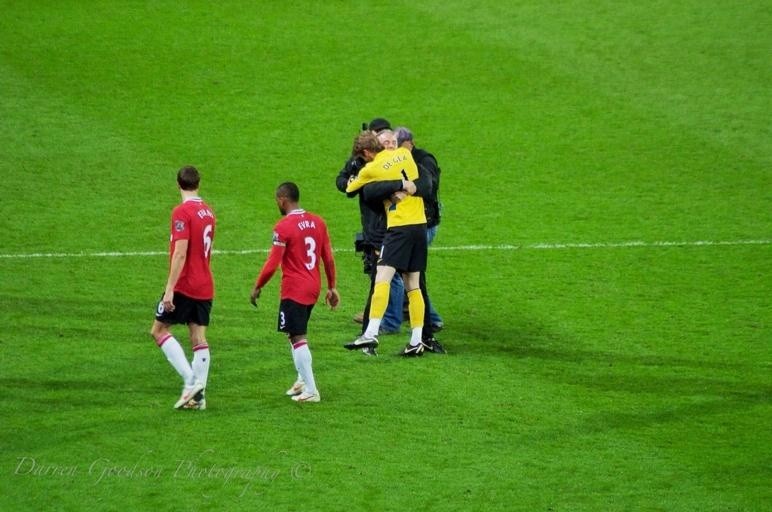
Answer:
[369,118,391,129]
[395,127,412,147]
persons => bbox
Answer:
[149,164,217,411]
[335,118,448,360]
[249,182,340,404]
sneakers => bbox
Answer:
[286,382,321,403]
[400,337,445,356]
[353,313,363,323]
[174,380,206,410]
[344,336,379,358]
